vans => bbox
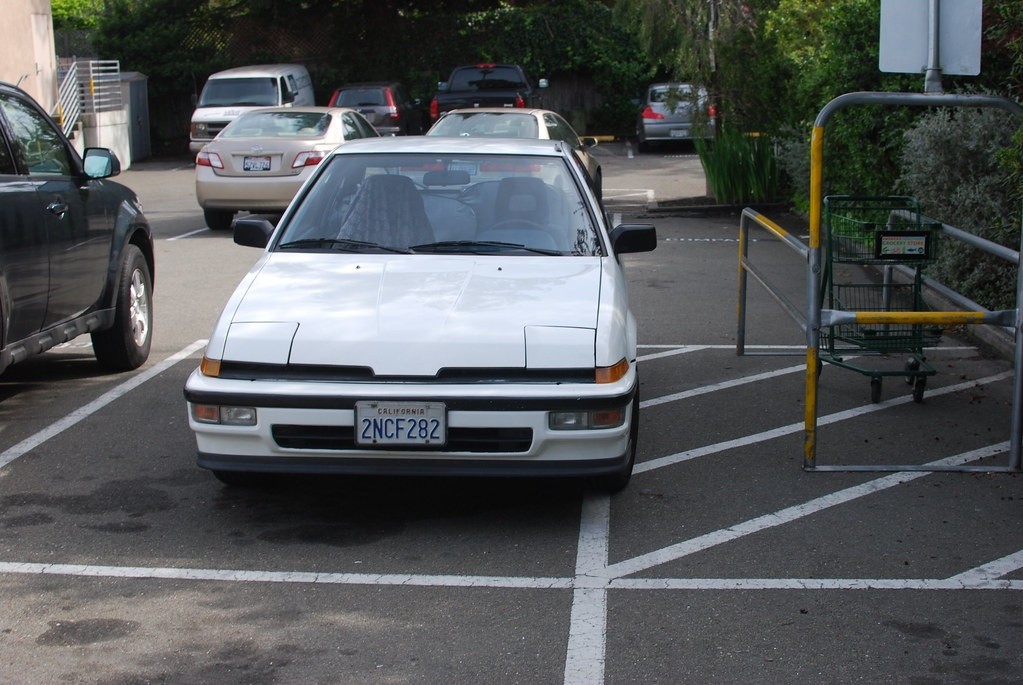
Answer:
[188,63,315,160]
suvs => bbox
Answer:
[430,63,549,124]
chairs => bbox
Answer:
[494,176,572,253]
[338,173,436,248]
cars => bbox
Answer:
[637,82,717,153]
[194,107,395,229]
[0,80,155,369]
[183,137,658,496]
[364,108,602,210]
[327,80,425,137]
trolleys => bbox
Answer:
[807,195,944,404]
